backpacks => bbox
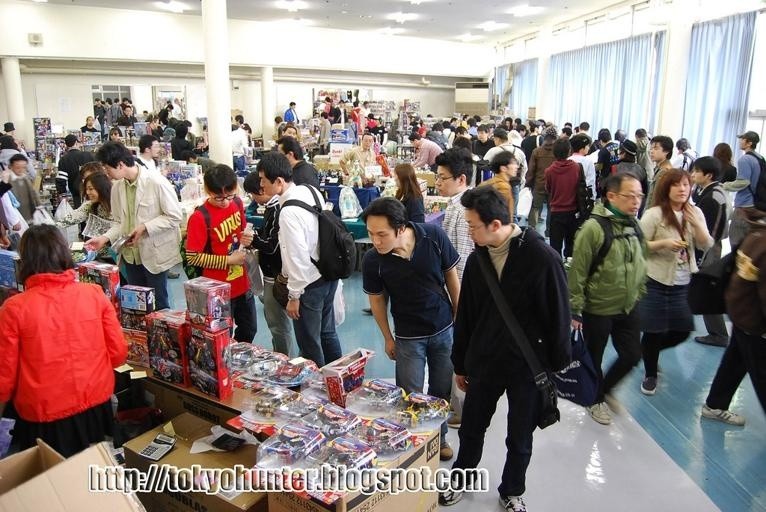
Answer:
[572,161,595,223]
[469,156,493,187]
[681,150,701,173]
[278,181,358,281]
[498,144,523,189]
[743,151,766,212]
[179,194,246,282]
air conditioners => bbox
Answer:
[453,81,494,118]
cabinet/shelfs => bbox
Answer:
[238,178,449,268]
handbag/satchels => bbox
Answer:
[550,323,603,408]
[534,374,563,430]
[336,184,364,220]
[514,187,534,220]
[686,270,729,315]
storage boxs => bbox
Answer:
[267,425,440,511]
[0,424,149,512]
[121,121,208,230]
[132,355,271,444]
[122,408,268,512]
[311,121,354,170]
[30,115,102,166]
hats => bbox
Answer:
[736,131,761,142]
[618,140,639,156]
[528,120,546,127]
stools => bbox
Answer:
[352,237,380,271]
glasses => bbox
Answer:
[613,191,646,200]
[206,191,237,201]
[433,172,457,181]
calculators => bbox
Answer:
[138,432,177,461]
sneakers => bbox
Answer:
[586,401,613,425]
[439,477,476,507]
[498,490,527,512]
[605,394,627,416]
[700,403,748,425]
[640,374,658,395]
[445,414,462,429]
[440,441,455,462]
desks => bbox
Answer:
[412,168,438,197]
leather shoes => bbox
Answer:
[362,307,374,315]
[694,332,729,348]
